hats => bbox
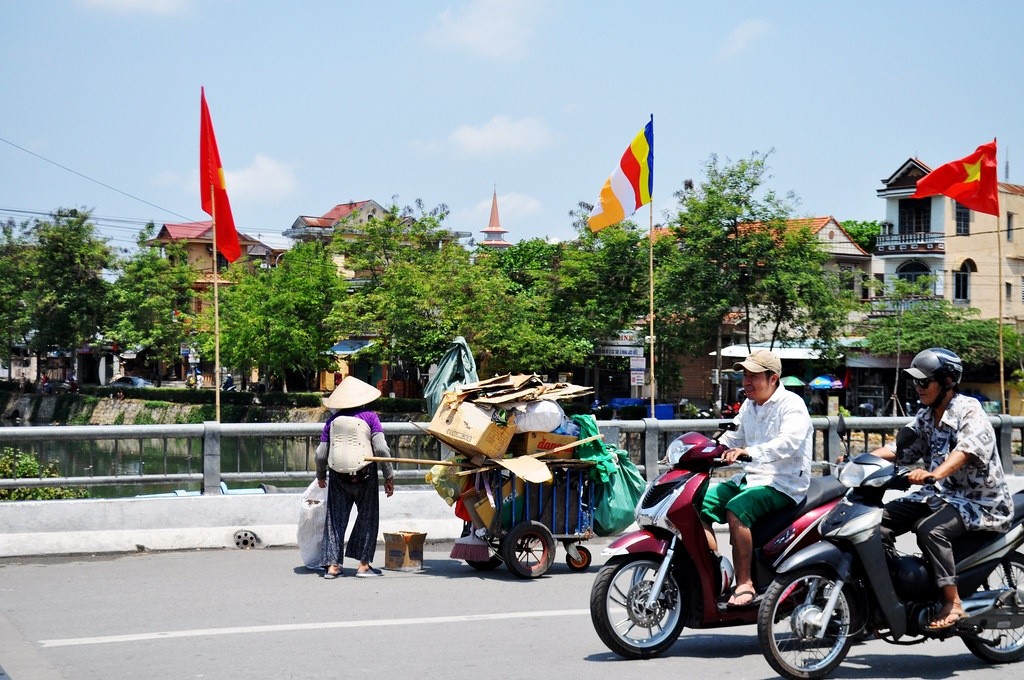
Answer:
[733,350,782,378]
[323,375,382,409]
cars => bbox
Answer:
[109,376,153,388]
[912,391,1001,416]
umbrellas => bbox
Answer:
[779,375,806,391]
[809,374,843,389]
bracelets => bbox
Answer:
[385,478,394,483]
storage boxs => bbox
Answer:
[383,529,427,572]
[427,391,518,461]
[512,433,578,463]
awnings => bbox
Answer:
[320,339,377,354]
[709,346,844,361]
[845,352,916,368]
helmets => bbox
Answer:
[710,550,734,599]
[227,374,232,377]
[901,348,963,390]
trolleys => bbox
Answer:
[461,460,597,579]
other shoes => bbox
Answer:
[356,567,383,577]
[324,569,344,579]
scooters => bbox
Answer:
[589,420,848,661]
[220,382,237,392]
[757,415,1024,680]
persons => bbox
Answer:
[835,348,1014,629]
[314,376,395,579]
[222,373,233,392]
[39,372,47,392]
[19,373,25,391]
[693,351,814,608]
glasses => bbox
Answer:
[911,378,934,389]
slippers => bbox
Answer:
[726,590,758,608]
[928,610,970,628]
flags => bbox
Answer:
[909,141,1000,216]
[588,114,653,233]
[200,90,242,264]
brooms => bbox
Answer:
[449,472,490,562]
